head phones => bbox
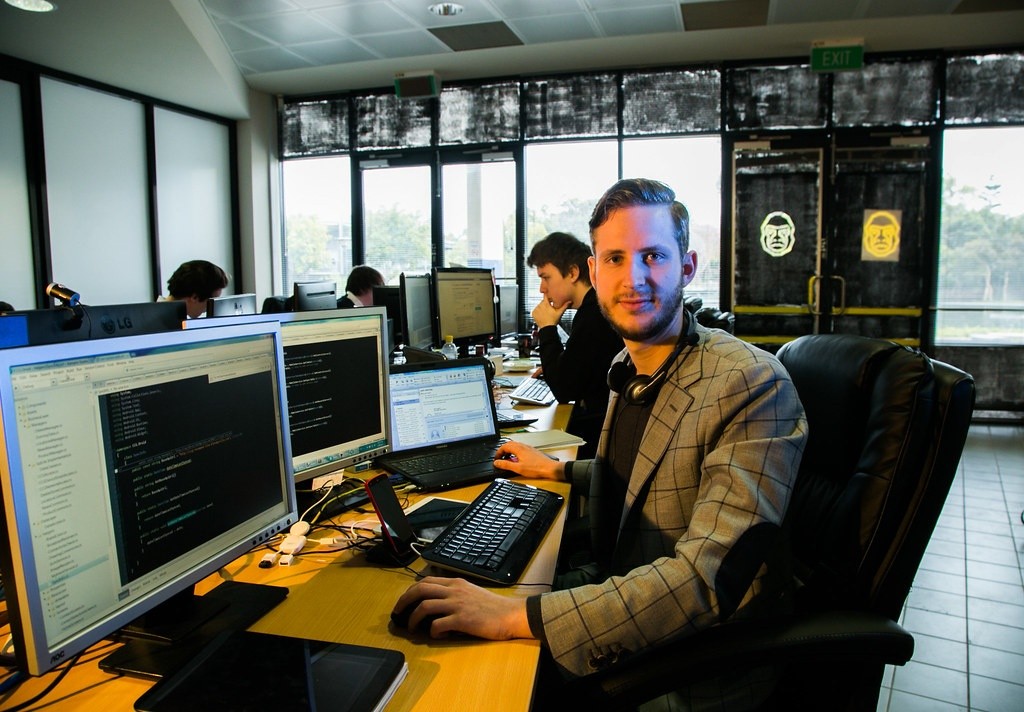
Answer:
[609,307,698,407]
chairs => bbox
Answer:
[682,293,703,314]
[694,307,736,335]
[599,332,976,712]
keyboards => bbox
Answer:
[422,478,566,590]
[508,376,556,406]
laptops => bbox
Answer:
[403,345,539,429]
[374,356,521,492]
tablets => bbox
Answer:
[373,496,470,545]
[132,629,405,712]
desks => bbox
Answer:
[0,434,578,711]
[426,326,574,434]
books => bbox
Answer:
[502,429,583,448]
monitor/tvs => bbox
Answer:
[0,266,519,677]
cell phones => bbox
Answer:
[364,473,420,555]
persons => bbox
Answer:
[390,178,809,712]
[156,260,229,319]
[337,266,385,310]
[526,232,624,461]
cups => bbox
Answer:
[518,333,532,358]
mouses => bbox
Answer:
[389,598,472,640]
[537,374,545,380]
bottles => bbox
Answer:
[441,336,458,360]
